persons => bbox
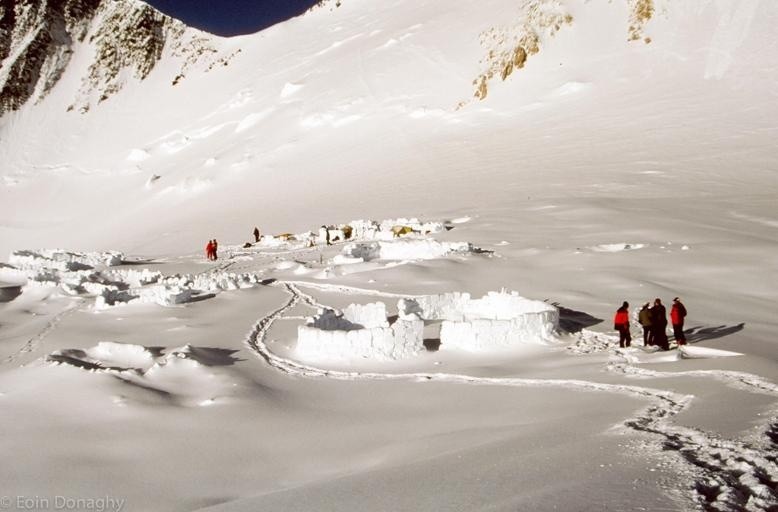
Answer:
[669,296,687,345]
[638,302,654,347]
[252,226,259,242]
[206,240,213,259]
[613,300,632,347]
[212,238,218,260]
[650,298,670,351]
